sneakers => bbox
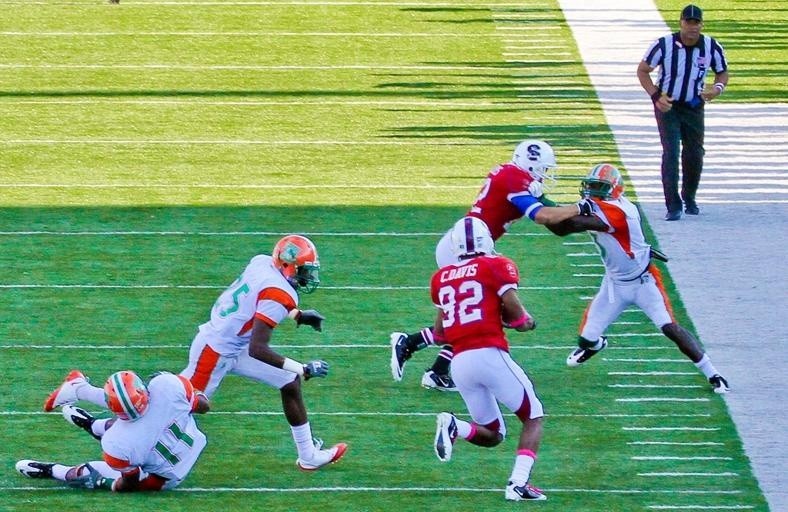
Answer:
[421,369,459,391]
[296,443,346,470]
[44,370,101,441]
[567,336,608,367]
[505,478,547,500]
[709,374,729,394]
[390,332,412,382]
[434,412,458,462]
[15,460,75,479]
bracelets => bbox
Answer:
[650,89,662,103]
[713,83,725,92]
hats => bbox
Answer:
[681,5,702,22]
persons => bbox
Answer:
[13,369,212,493]
[390,138,558,393]
[40,233,348,471]
[526,163,733,396]
[634,0,730,222]
[428,216,548,503]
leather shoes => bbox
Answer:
[681,190,699,214]
[665,210,682,220]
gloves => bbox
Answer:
[303,360,328,378]
[297,310,325,331]
[576,198,598,215]
[528,180,542,197]
[68,463,116,492]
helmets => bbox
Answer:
[272,235,320,294]
[452,217,494,261]
[513,139,556,191]
[104,371,151,421]
[579,164,624,201]
